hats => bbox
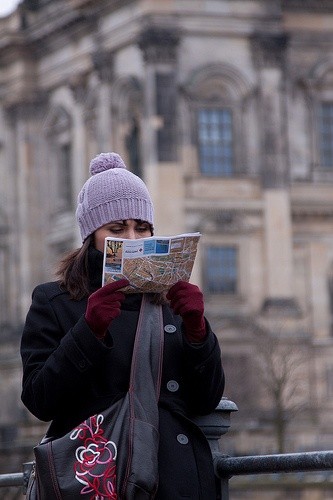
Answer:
[75,152,153,240]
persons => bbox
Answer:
[20,152,228,500]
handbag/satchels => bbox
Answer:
[22,392,158,500]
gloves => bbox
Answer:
[166,281,207,341]
[83,278,129,341]
